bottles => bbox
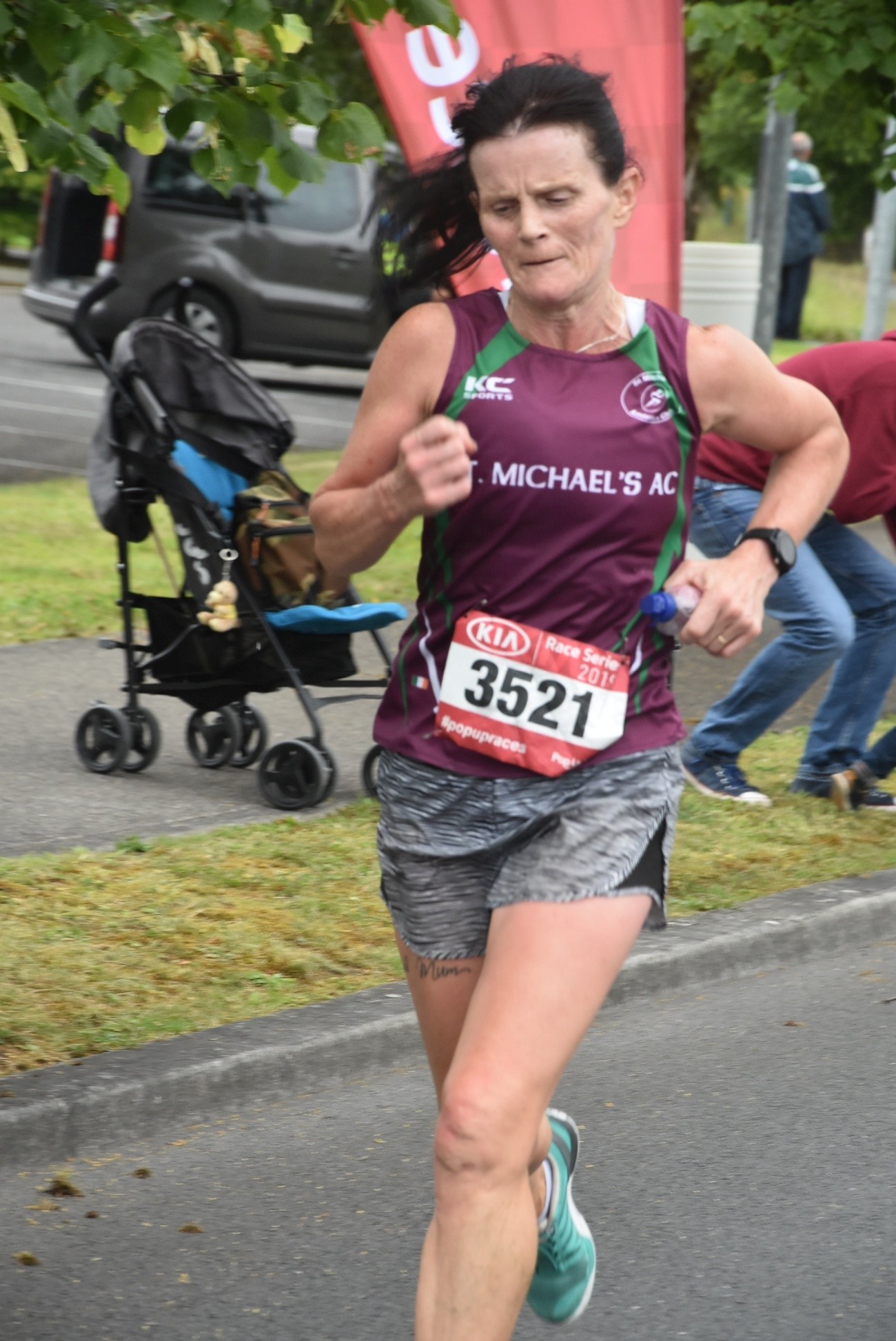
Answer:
[639,583,705,633]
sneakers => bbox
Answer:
[788,778,832,798]
[527,1107,597,1326]
[834,771,867,813]
[863,791,896,809]
[678,751,773,808]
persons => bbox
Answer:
[303,53,851,1341]
[687,329,895,811]
[830,721,895,811]
[776,131,834,340]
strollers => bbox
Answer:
[66,272,418,812]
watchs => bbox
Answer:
[733,527,799,578]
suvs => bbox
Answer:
[18,94,458,368]
